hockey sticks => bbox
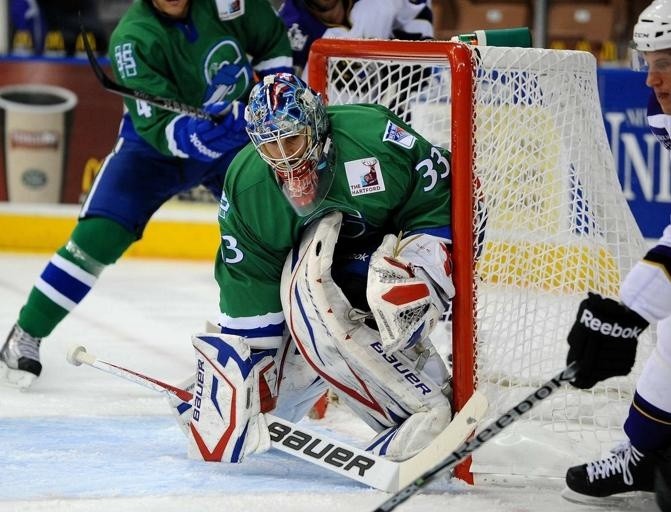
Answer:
[78,11,221,125]
[66,344,489,492]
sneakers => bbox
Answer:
[0,322,41,376]
[566,441,655,497]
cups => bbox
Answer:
[0,84,78,203]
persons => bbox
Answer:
[565,0,671,510]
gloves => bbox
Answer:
[567,292,649,389]
[174,102,250,162]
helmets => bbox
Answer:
[632,0,671,72]
[245,73,329,181]
